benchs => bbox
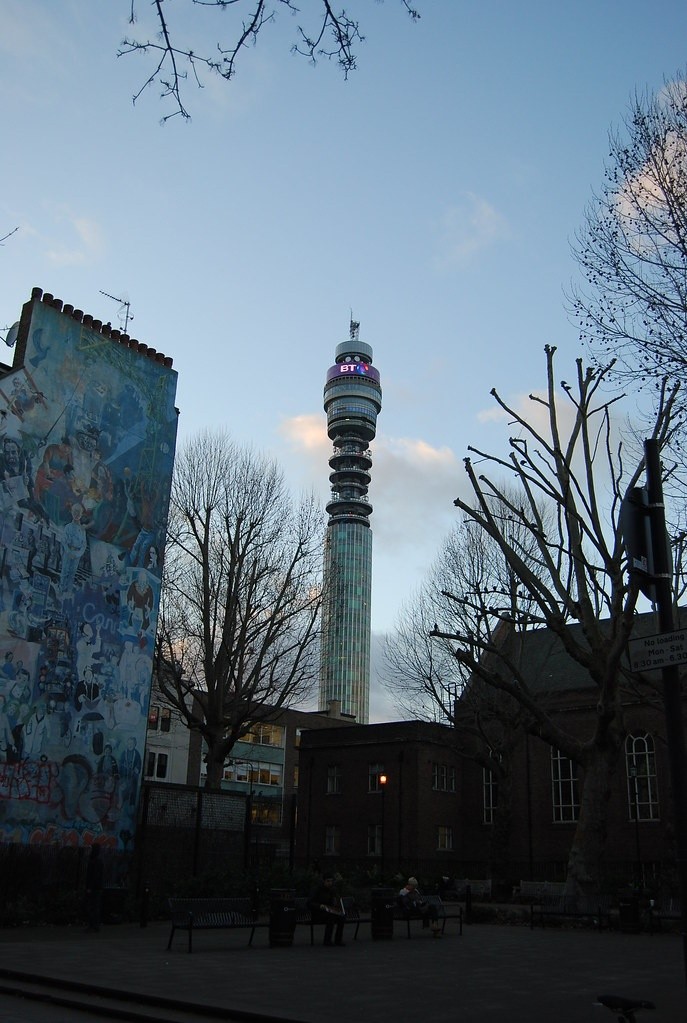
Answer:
[398,895,463,936]
[433,877,492,902]
[531,895,611,934]
[166,898,273,953]
[295,897,377,945]
[512,880,566,905]
[648,894,683,936]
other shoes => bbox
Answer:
[432,933,442,938]
[431,924,441,931]
[323,941,336,946]
[335,942,346,946]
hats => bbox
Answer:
[323,873,335,880]
[408,877,418,885]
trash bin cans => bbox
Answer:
[370,887,395,940]
[269,887,297,945]
[619,894,642,935]
[84,887,100,915]
[101,886,129,924]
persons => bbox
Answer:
[400,877,444,939]
[307,873,347,947]
